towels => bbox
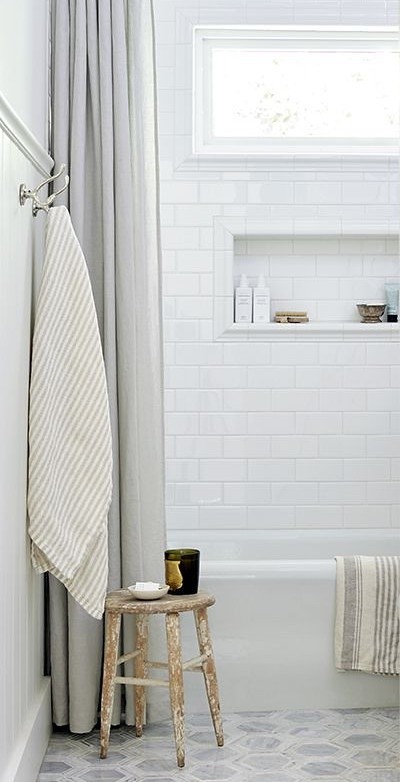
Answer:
[28,205,117,623]
[333,555,399,678]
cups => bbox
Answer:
[164,547,200,596]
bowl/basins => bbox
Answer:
[127,584,170,600]
[356,303,387,323]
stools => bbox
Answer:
[99,588,224,768]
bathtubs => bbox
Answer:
[172,528,400,709]
